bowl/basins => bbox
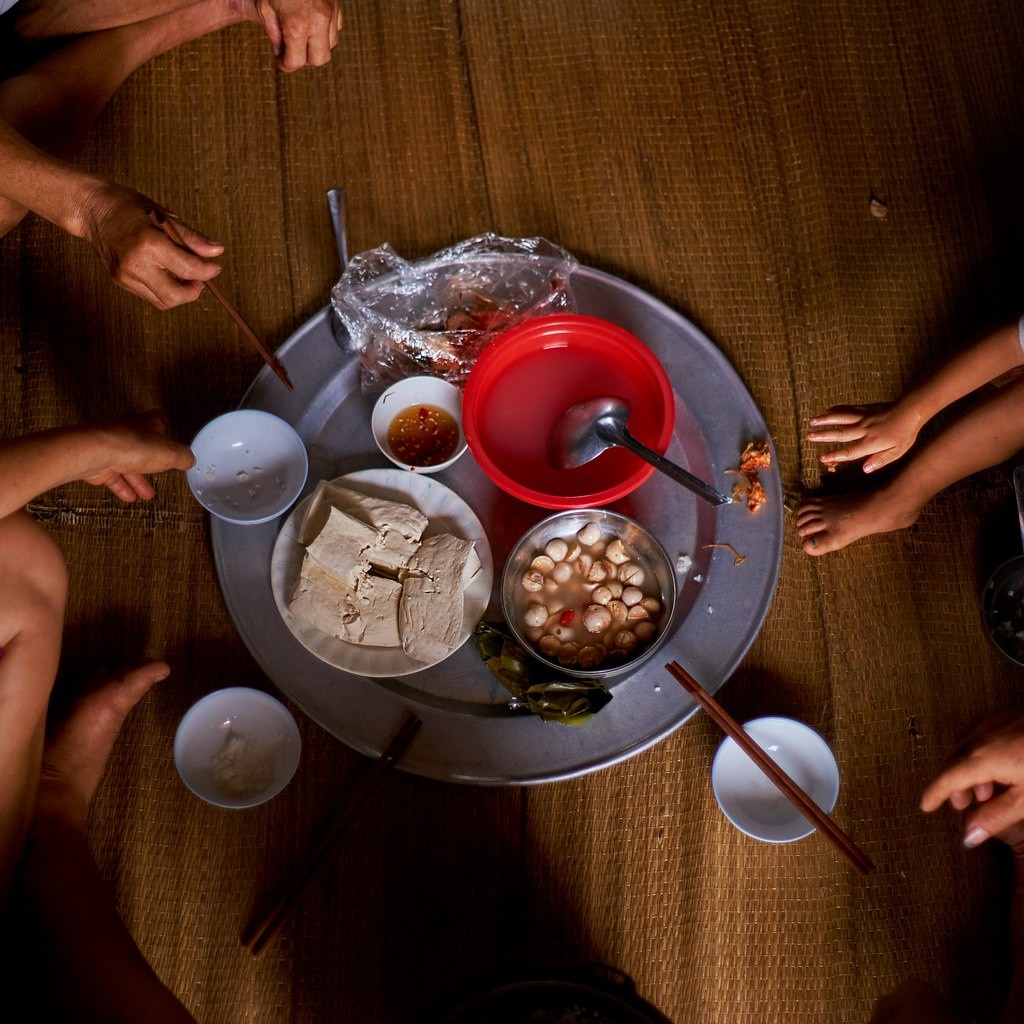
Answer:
[462,314,675,510]
[371,375,469,474]
[711,717,840,843]
[186,410,308,525]
[500,508,678,680]
[173,686,302,809]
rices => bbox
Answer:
[207,734,277,801]
[197,463,286,508]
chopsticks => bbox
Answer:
[664,660,875,877]
[241,713,422,957]
[146,209,294,394]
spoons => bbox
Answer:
[327,188,371,354]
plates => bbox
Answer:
[269,468,494,678]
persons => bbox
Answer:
[1,413,344,1024]
[0,1,344,310]
[798,313,1024,849]
